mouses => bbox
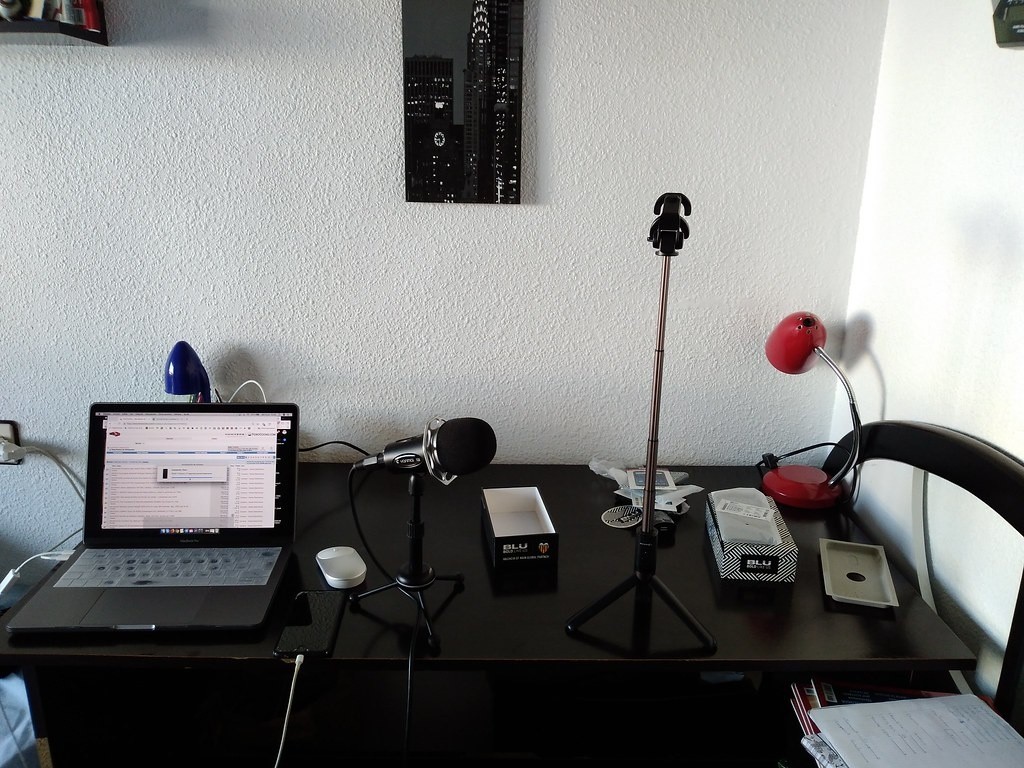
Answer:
[316,546,367,588]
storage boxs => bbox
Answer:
[479,483,560,574]
[705,492,799,582]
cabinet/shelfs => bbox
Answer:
[0,461,978,768]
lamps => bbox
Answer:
[762,311,861,509]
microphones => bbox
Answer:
[353,416,497,485]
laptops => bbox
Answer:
[7,401,299,634]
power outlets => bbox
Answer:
[0,419,24,466]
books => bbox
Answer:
[22,0,101,32]
[790,676,1023,768]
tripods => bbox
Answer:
[564,193,720,653]
[348,472,465,645]
